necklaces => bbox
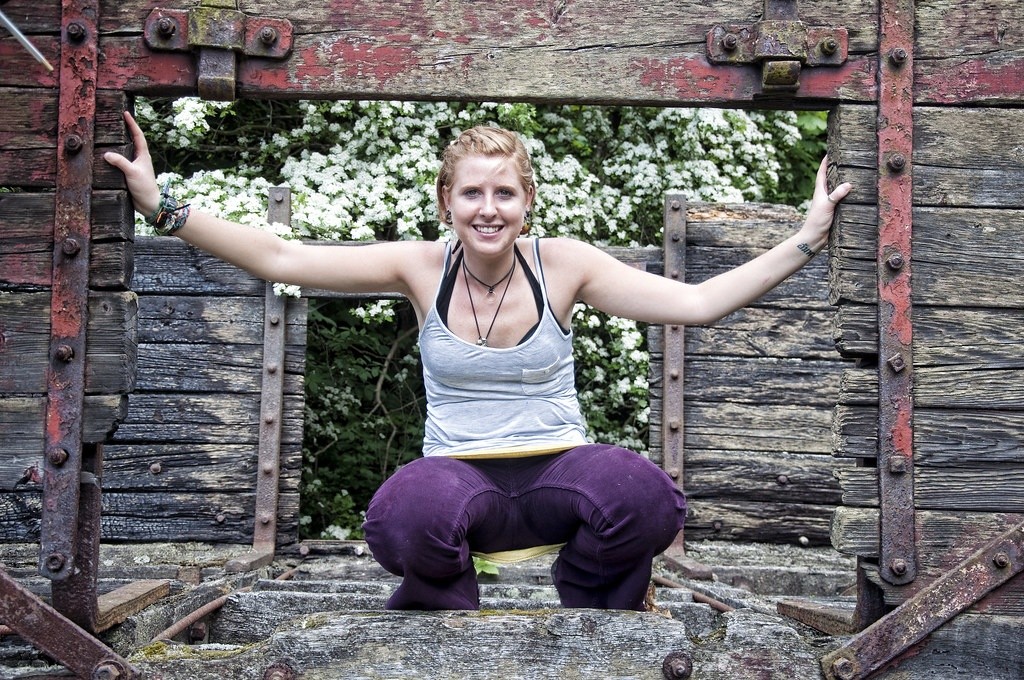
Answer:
[460,247,516,349]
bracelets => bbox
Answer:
[143,175,193,238]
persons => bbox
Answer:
[103,106,854,614]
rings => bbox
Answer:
[828,195,838,205]
